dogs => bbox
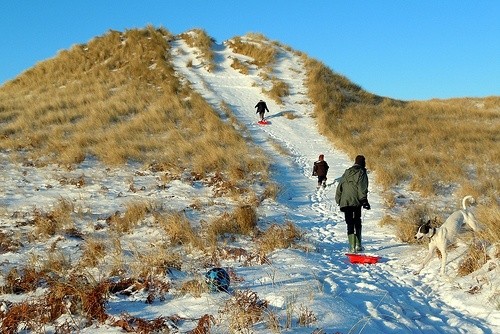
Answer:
[413,195,482,275]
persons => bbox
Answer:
[313,154,329,187]
[335,154,371,253]
[254,98,270,121]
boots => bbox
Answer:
[348,234,356,253]
[356,235,364,251]
